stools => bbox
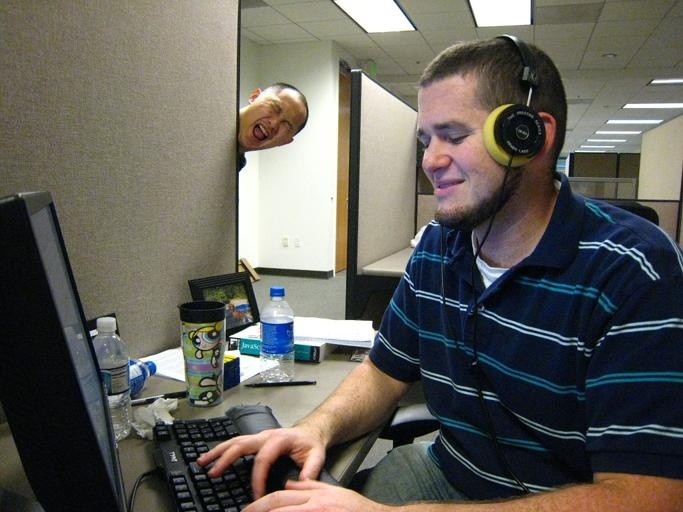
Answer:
[349,402,440,495]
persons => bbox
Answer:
[238,82,309,173]
[197,38,683,512]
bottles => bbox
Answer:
[125,357,157,394]
[91,314,133,442]
[258,286,296,382]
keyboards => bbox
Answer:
[152,405,342,512]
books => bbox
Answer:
[228,315,374,362]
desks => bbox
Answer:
[0,315,398,512]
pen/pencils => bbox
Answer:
[131,391,189,406]
[243,381,317,387]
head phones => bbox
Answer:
[484,34,545,168]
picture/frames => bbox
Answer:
[188,270,260,341]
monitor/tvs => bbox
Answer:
[0,189,131,512]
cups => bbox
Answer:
[178,302,226,407]
